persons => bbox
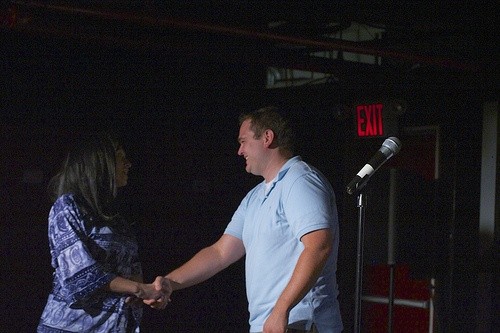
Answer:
[148,105,344,333]
[36,130,172,333]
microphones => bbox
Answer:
[346,136,401,195]
